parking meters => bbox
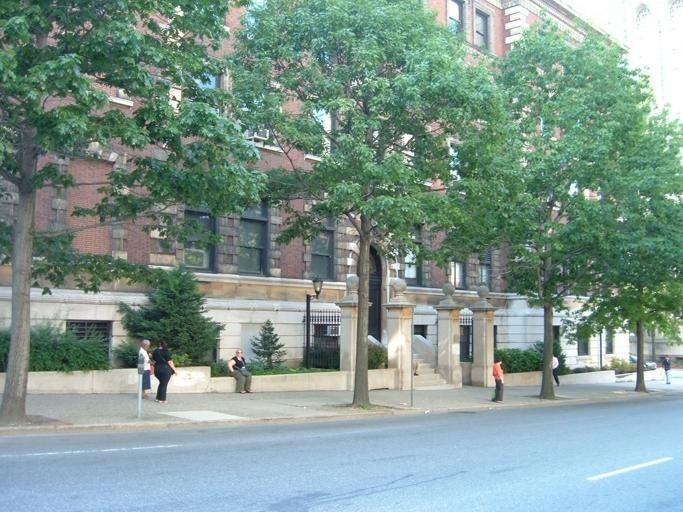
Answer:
[137,354,145,418]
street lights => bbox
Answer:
[305,275,322,369]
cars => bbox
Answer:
[629,354,656,372]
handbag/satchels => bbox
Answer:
[167,365,174,375]
[238,366,249,377]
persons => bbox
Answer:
[152,339,179,403]
[661,355,672,384]
[138,339,155,400]
[490,359,506,403]
[551,353,561,387]
[225,348,253,394]
